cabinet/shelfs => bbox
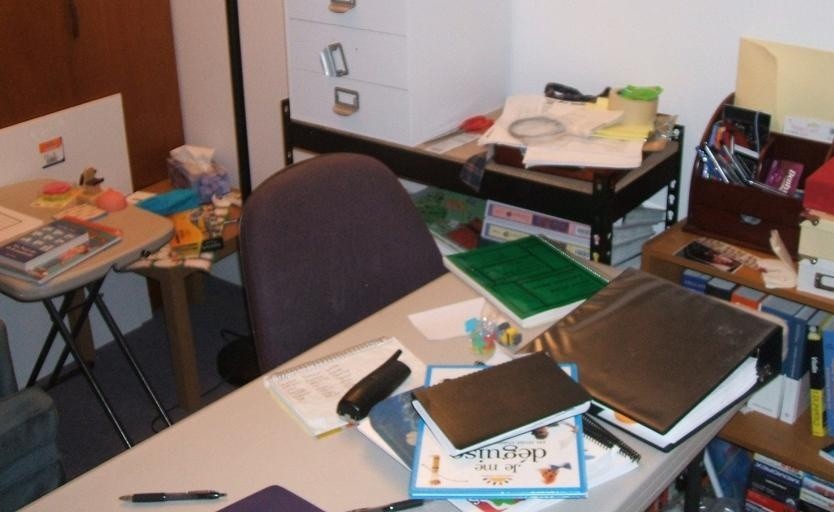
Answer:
[633,215,832,512]
[167,0,322,291]
[283,0,513,143]
[1,0,187,193]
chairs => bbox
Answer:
[237,149,448,377]
[0,319,65,512]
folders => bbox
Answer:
[513,267,784,453]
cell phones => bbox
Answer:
[337,349,411,421]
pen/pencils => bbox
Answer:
[349,499,425,512]
[221,218,238,223]
[695,129,788,196]
[120,492,225,502]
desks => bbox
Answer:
[277,91,686,264]
[0,177,179,455]
[8,251,767,512]
[120,178,252,416]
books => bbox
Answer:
[0,215,123,284]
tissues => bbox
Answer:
[165,144,232,204]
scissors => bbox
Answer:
[424,117,491,144]
